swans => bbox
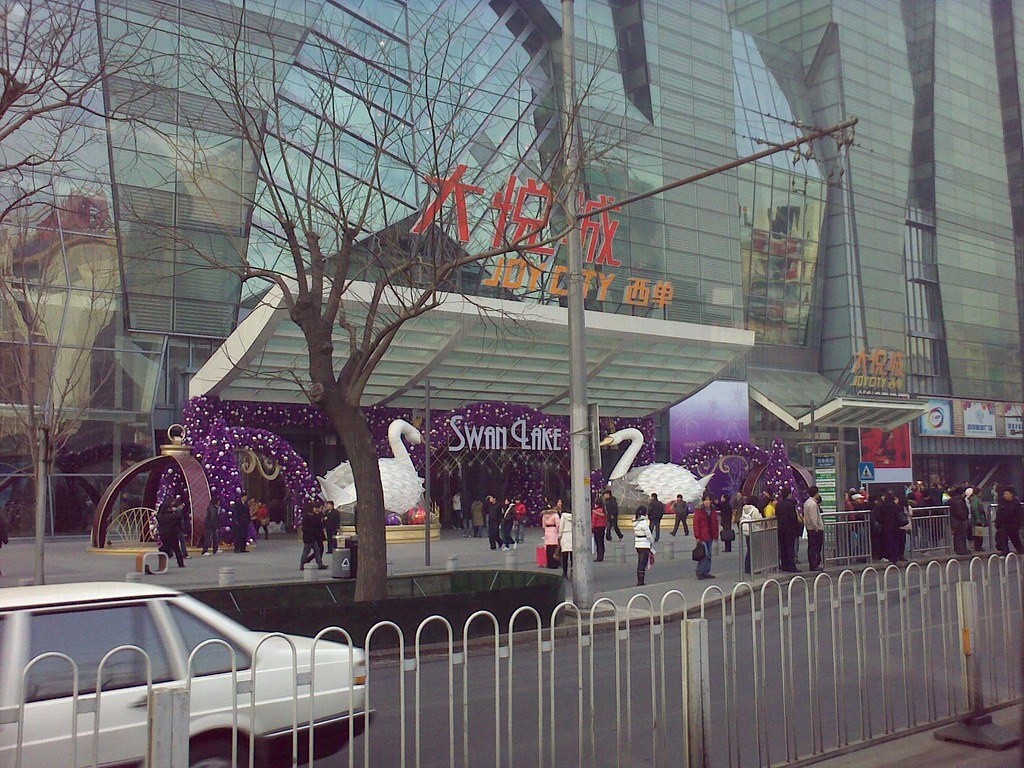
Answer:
[600,428,715,514]
[316,419,437,515]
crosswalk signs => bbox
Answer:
[857,462,875,483]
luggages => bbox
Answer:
[536,537,547,568]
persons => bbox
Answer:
[669,494,689,536]
[300,501,341,570]
[234,493,270,552]
[453,491,527,551]
[202,498,222,556]
[541,498,572,579]
[803,486,824,571]
[845,482,988,566]
[632,506,654,586]
[592,498,607,562]
[693,493,732,580]
[647,493,663,542]
[0,516,9,577]
[353,505,358,533]
[735,487,802,572]
[994,482,1024,559]
[156,499,192,567]
[604,490,624,542]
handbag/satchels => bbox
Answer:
[261,517,270,526]
[553,544,561,560]
[972,524,989,537]
[692,543,706,561]
[647,548,656,569]
[720,527,735,542]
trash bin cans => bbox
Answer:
[331,535,359,579]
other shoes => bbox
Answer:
[300,566,304,570]
[214,550,223,555]
[502,547,510,551]
[698,572,703,579]
[704,572,714,578]
[814,567,823,571]
[203,552,211,556]
[499,541,504,548]
[513,542,517,549]
[490,548,496,550]
[594,559,601,562]
[788,568,801,572]
[318,566,328,569]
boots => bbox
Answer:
[637,570,645,586]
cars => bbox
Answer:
[0,581,375,768]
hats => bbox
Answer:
[852,494,864,500]
